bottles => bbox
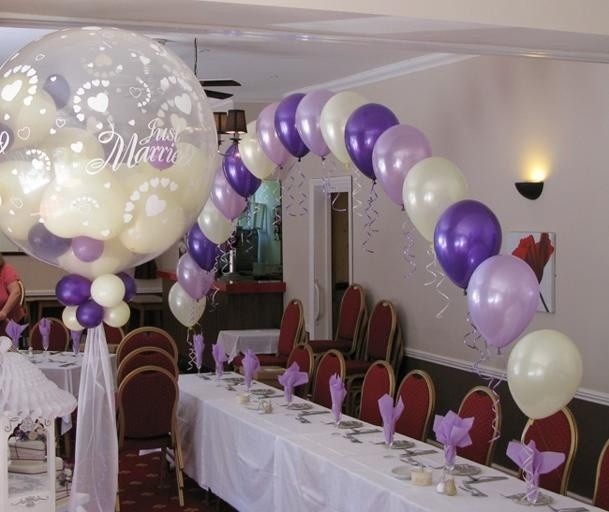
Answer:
[435,472,457,495]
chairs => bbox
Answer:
[0,312,189,512]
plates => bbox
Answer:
[250,388,274,395]
[451,462,479,474]
[338,419,360,428]
[223,376,246,385]
[288,402,310,410]
[393,466,414,478]
[392,441,415,449]
[525,493,551,504]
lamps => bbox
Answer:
[513,182,543,200]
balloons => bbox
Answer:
[318,91,368,164]
[372,123,432,209]
[432,199,502,291]
[465,253,541,350]
[343,101,399,181]
[506,327,585,422]
[198,93,328,246]
[1,22,217,331]
[400,156,468,244]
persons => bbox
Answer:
[0,255,26,350]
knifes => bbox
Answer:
[464,479,510,484]
[465,484,487,496]
